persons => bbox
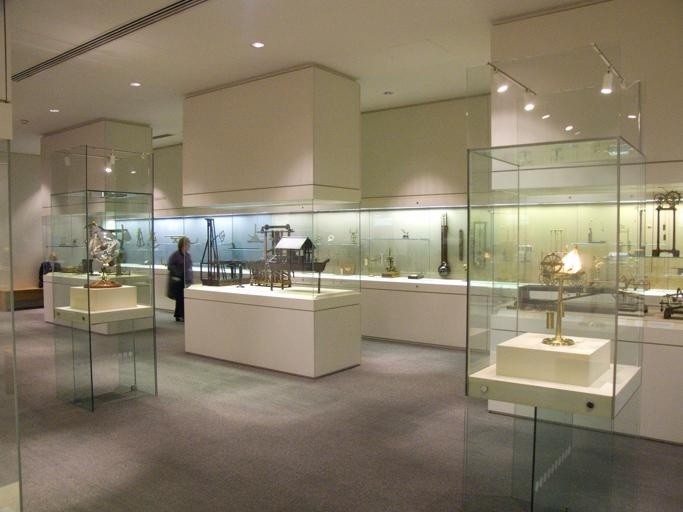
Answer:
[166,237,192,322]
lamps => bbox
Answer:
[601,70,614,95]
[487,61,537,112]
